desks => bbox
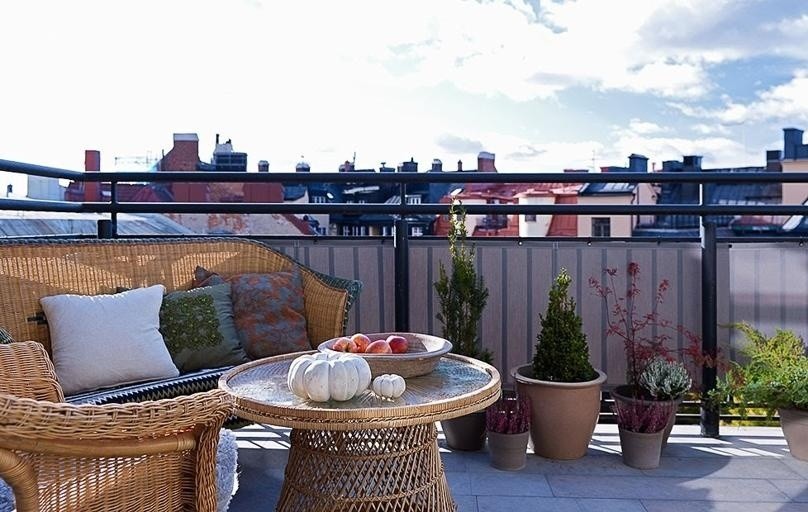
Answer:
[214,343,503,512]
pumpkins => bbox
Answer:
[372,374,406,398]
[287,351,372,402]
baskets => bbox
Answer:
[318,333,453,379]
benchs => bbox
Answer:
[0,232,363,438]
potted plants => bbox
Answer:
[584,257,730,455]
[427,187,503,454]
[693,315,808,467]
[507,266,608,461]
[597,388,679,471]
[480,384,537,473]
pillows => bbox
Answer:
[0,327,17,348]
[113,278,254,377]
[191,258,320,362]
[33,281,182,396]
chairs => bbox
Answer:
[0,338,236,512]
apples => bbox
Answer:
[364,340,393,354]
[387,335,409,354]
[351,333,371,353]
[334,337,357,353]
